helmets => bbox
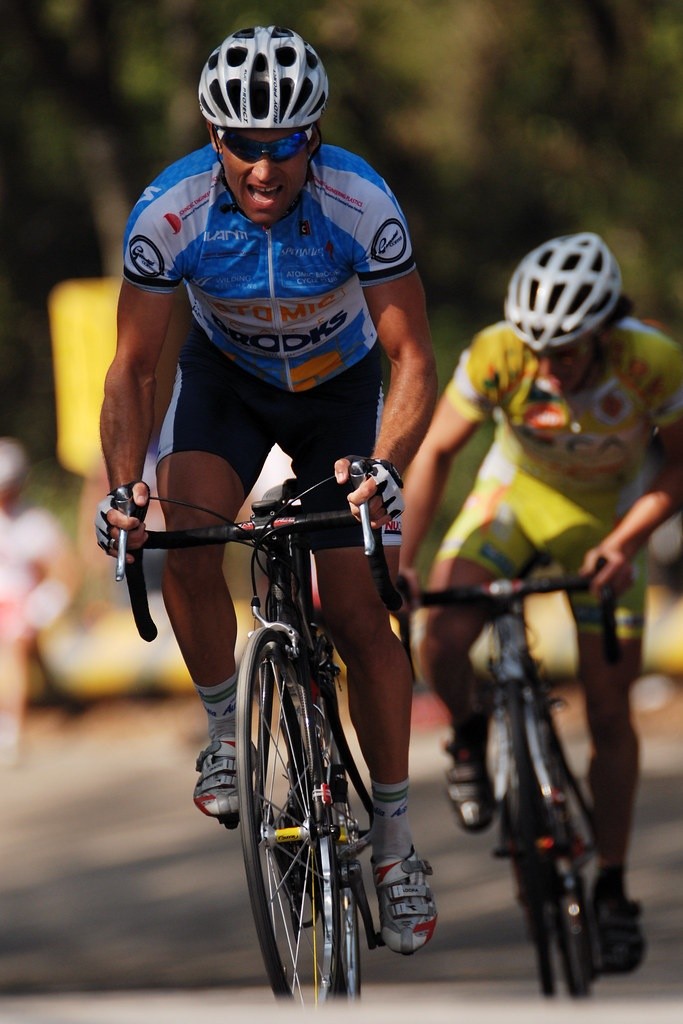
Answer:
[197,25,329,128]
[503,231,622,355]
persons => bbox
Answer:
[394,232,683,978]
[0,438,77,762]
[93,22,439,955]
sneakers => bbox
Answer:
[370,842,438,954]
[193,732,257,817]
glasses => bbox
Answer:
[220,130,309,163]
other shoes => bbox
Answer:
[445,759,494,833]
[590,885,647,971]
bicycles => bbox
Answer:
[390,553,643,1003]
[112,454,416,1010]
